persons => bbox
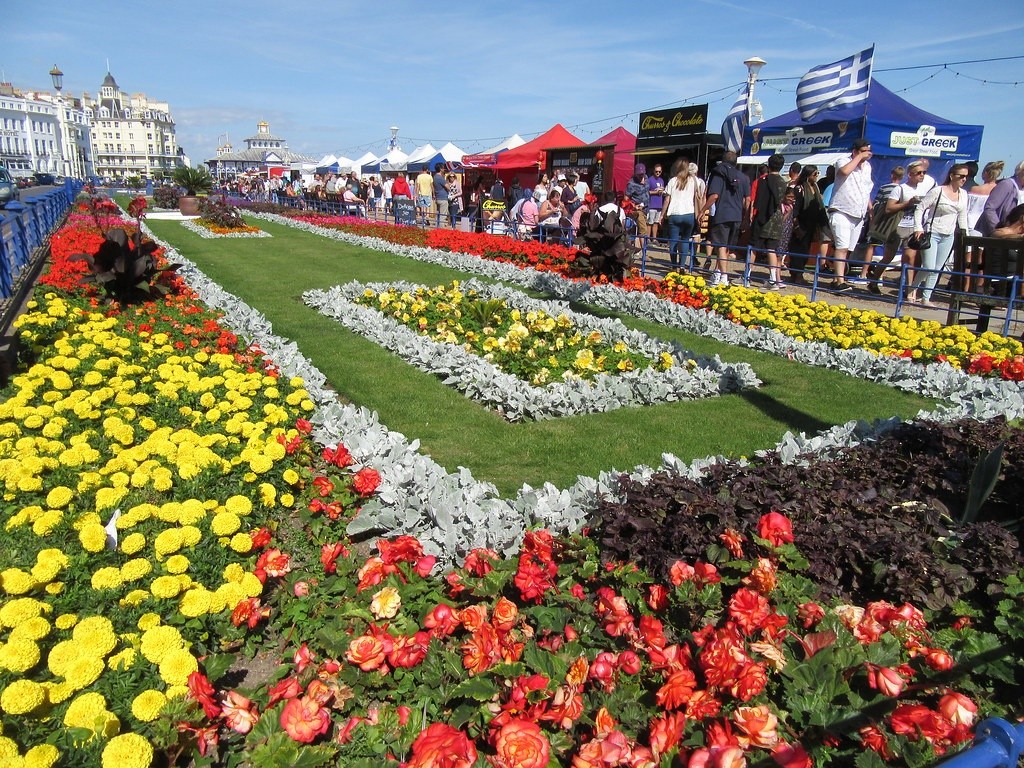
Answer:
[217,138,1024,309]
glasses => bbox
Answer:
[656,171,662,173]
[911,170,926,175]
[953,174,969,179]
[812,172,819,176]
[449,176,455,178]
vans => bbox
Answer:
[33,173,56,186]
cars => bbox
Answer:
[24,176,40,186]
[0,166,20,205]
[13,176,26,189]
[54,175,66,186]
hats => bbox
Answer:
[635,163,646,174]
[558,174,567,181]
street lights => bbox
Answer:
[390,126,399,147]
[49,64,75,208]
[284,146,290,165]
[743,56,766,125]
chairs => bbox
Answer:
[503,210,534,241]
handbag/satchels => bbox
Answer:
[907,231,931,249]
[819,206,827,226]
[868,203,904,241]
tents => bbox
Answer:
[742,75,985,272]
[306,123,638,205]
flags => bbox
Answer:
[796,46,876,124]
[721,81,747,155]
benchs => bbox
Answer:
[276,191,304,209]
[303,188,365,217]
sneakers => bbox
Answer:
[731,276,750,287]
[760,280,778,291]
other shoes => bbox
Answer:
[920,299,935,308]
[867,284,880,295]
[641,254,651,261]
[830,282,837,293]
[907,289,917,303]
[977,286,995,293]
[652,240,662,247]
[791,272,808,283]
[669,265,685,275]
[834,283,852,292]
[710,269,728,288]
[776,279,787,288]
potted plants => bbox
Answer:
[171,164,214,215]
